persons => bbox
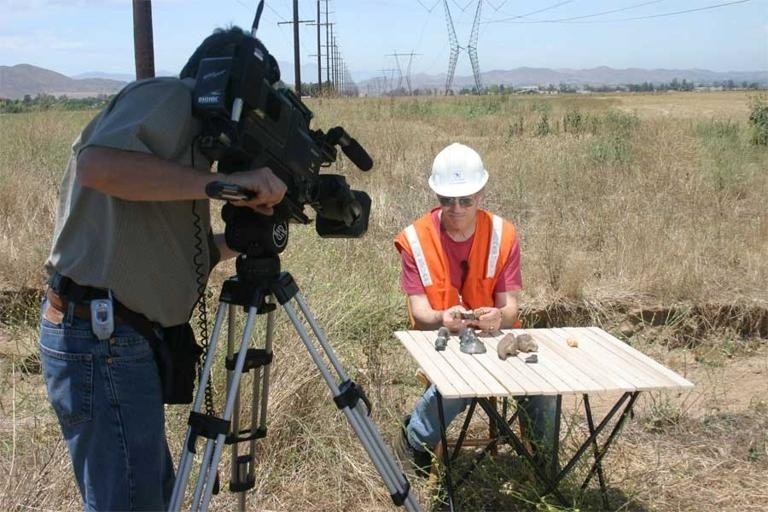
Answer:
[392,142,563,479]
[37,23,290,512]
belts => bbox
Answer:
[45,285,137,326]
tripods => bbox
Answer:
[166,251,425,512]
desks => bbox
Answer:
[393,326,695,512]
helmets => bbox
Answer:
[428,141,489,198]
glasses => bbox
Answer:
[436,196,475,207]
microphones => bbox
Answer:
[435,326,450,350]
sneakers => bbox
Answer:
[390,414,432,479]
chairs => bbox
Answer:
[406,296,537,489]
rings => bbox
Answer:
[489,326,494,330]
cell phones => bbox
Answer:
[462,312,475,320]
[89,288,115,341]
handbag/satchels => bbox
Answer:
[155,320,203,406]
